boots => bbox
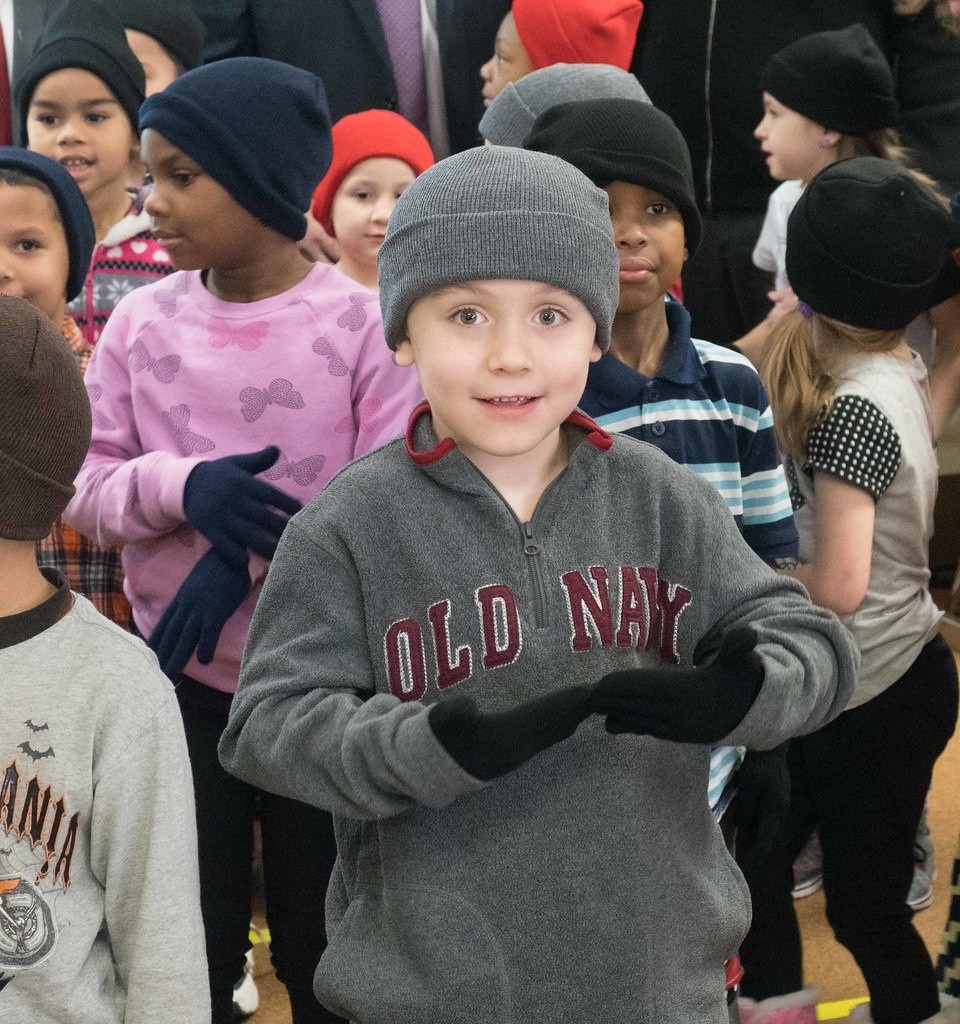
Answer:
[737,983,818,1024]
[847,995,960,1024]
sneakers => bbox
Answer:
[789,832,825,898]
[231,923,273,1019]
[903,851,937,911]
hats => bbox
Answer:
[479,62,655,149]
[763,25,898,138]
[789,155,952,330]
[311,112,435,238]
[512,0,642,74]
[376,145,618,356]
[12,0,145,135]
[522,98,702,265]
[0,293,88,540]
[0,145,96,301]
[138,56,333,240]
[107,0,208,74]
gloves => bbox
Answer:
[593,625,766,745]
[146,545,250,682]
[183,445,302,570]
[423,680,596,784]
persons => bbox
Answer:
[0,0,435,945]
[725,23,960,1024]
[62,57,426,1024]
[525,97,798,1023]
[478,1,653,149]
[218,145,859,1024]
[0,293,212,1024]
